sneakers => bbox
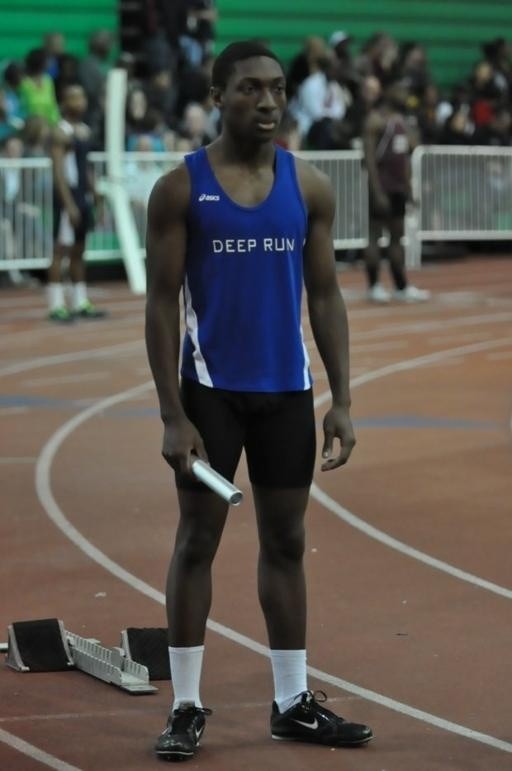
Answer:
[271,692,373,745]
[155,701,207,759]
[369,283,428,302]
[48,302,104,322]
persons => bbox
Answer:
[120,0,215,66]
[0,30,512,289]
[364,74,431,305]
[144,41,374,759]
[46,82,106,321]
